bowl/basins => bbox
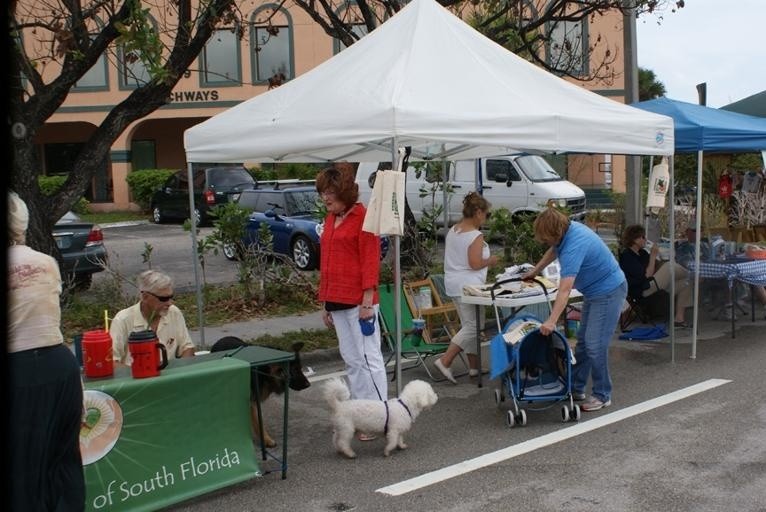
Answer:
[744,246,766,260]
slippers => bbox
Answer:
[674,320,692,328]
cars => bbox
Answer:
[673,170,697,204]
[51,212,109,291]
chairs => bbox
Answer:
[104,309,113,333]
[620,276,669,333]
[402,278,487,344]
[377,283,470,383]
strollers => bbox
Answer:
[491,278,583,428]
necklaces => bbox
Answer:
[335,204,355,219]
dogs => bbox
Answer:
[209,336,311,448]
[320,376,439,458]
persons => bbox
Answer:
[8,191,86,512]
[619,225,693,328]
[676,219,740,321]
[109,270,195,370]
[433,192,498,386]
[749,284,766,320]
[316,164,389,442]
[520,207,628,412]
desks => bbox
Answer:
[461,289,586,402]
[687,253,766,339]
[78,345,296,512]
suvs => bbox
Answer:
[221,179,391,274]
[150,163,258,226]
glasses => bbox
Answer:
[141,291,173,301]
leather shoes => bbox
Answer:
[433,358,458,385]
[469,368,489,377]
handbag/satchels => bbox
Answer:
[362,148,405,237]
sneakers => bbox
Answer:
[581,396,611,411]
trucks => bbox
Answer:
[354,150,587,242]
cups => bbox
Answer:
[80,330,113,378]
[413,319,426,345]
[128,330,167,378]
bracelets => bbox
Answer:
[360,306,374,310]
